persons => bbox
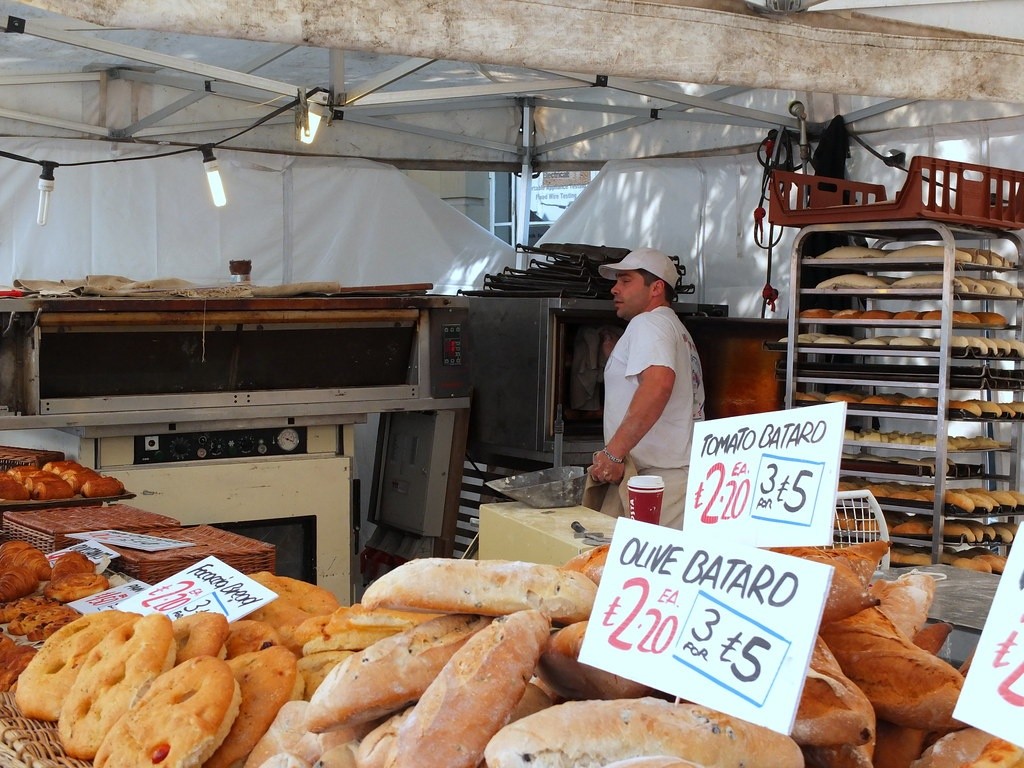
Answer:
[592,247,704,530]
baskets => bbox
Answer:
[76,524,276,586]
[0,692,95,768]
[0,446,65,471]
[3,504,180,555]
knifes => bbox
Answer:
[571,521,612,543]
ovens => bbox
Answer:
[459,292,730,467]
[0,300,471,612]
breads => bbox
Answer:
[0,540,340,768]
[234,539,1024,768]
[0,459,124,501]
[778,238,1023,575]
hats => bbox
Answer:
[599,247,679,289]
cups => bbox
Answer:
[627,475,664,525]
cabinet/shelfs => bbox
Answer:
[783,220,1024,572]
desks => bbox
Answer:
[477,502,623,566]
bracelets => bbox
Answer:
[603,446,626,463]
